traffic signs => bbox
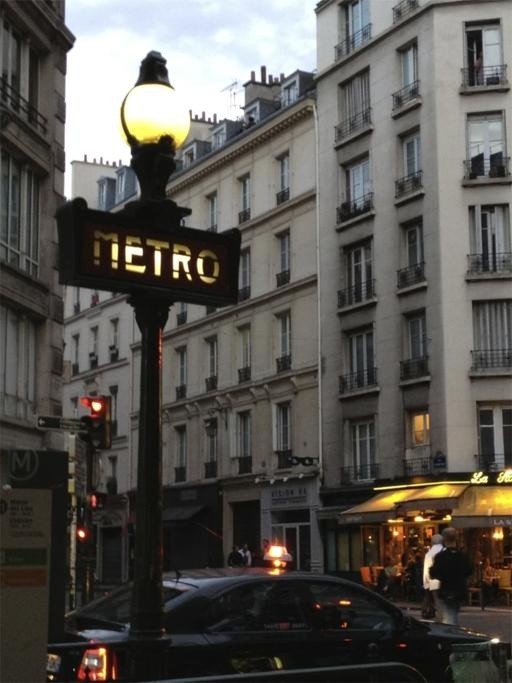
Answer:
[35,414,90,434]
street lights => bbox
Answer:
[118,49,192,679]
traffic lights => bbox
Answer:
[86,490,106,510]
[78,394,109,445]
[77,520,90,540]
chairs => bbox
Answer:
[359,566,386,598]
[468,568,512,606]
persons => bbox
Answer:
[225,523,511,627]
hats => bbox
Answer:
[442,528,457,548]
[431,534,442,544]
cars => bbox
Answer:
[43,568,511,681]
